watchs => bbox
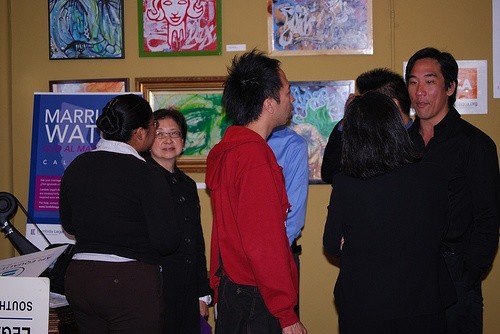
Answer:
[199,295,212,305]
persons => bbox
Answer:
[320,47,500,334]
[58,47,308,334]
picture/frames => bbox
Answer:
[48,0,125,60]
[49,78,130,92]
[137,0,223,57]
[288,80,355,184]
[135,76,231,173]
[266,0,374,57]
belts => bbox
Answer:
[291,244,301,254]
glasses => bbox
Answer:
[148,120,159,129]
[155,128,183,138]
